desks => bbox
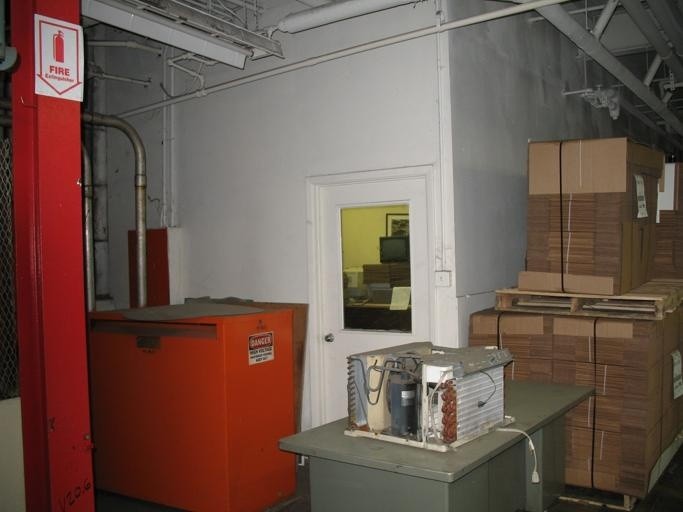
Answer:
[278,380,597,511]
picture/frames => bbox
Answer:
[384,213,410,238]
